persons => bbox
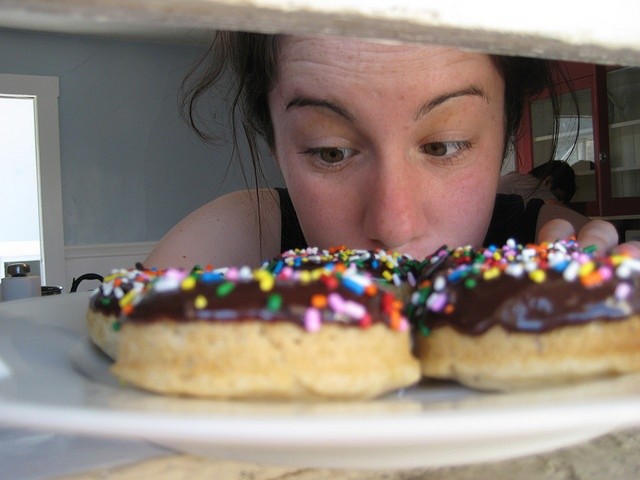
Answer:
[132,27,639,272]
[496,158,576,207]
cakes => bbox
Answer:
[83,258,196,363]
[110,259,419,398]
[414,237,639,392]
[273,244,421,295]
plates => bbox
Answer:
[1,255,640,471]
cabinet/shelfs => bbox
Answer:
[514,62,639,244]
